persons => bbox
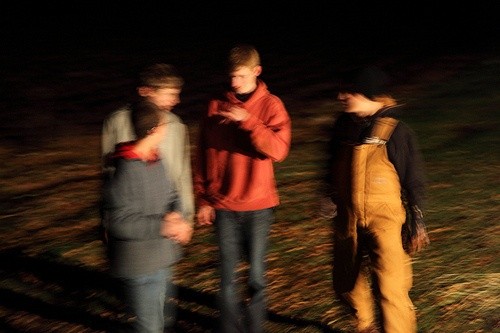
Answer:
[95,59,195,245]
[191,45,294,333]
[100,101,194,333]
[316,51,430,333]
